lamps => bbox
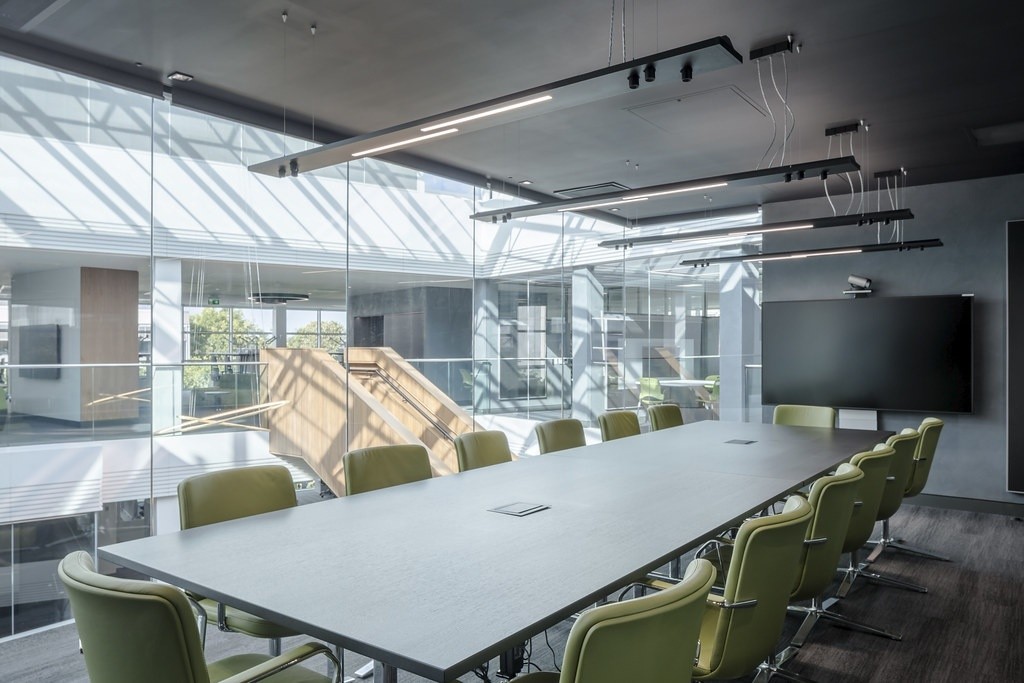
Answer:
[470,36,860,224]
[680,166,943,268]
[598,120,914,249]
[247,0,744,179]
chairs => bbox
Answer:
[57,374,952,683]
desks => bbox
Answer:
[636,380,715,387]
[99,419,897,683]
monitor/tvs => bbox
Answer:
[761,293,973,415]
